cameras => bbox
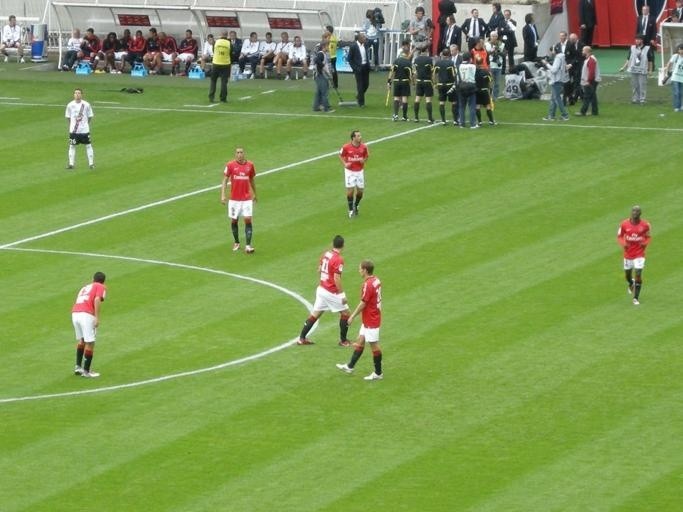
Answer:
[661,71,672,84]
[534,61,544,68]
[492,56,501,66]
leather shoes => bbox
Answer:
[360,104,366,108]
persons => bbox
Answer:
[71,271,107,377]
[238,32,260,80]
[200,33,216,72]
[153,32,179,74]
[616,205,653,305]
[433,48,457,125]
[471,36,490,73]
[171,29,199,76]
[541,47,570,122]
[91,31,120,69]
[0,15,25,63]
[667,43,683,111]
[554,31,575,105]
[285,36,308,80]
[461,9,487,52]
[437,0,457,55]
[623,35,653,104]
[108,28,132,74]
[569,33,585,102]
[228,31,242,64]
[501,10,518,74]
[264,32,292,80]
[672,1,683,23]
[363,7,385,65]
[522,13,540,61]
[118,30,146,74]
[321,25,339,89]
[297,235,356,347]
[62,29,83,71]
[413,47,434,123]
[338,131,369,217]
[143,28,158,69]
[410,6,432,56]
[77,28,100,62]
[456,53,480,129]
[334,258,385,381]
[65,89,95,169]
[387,46,413,121]
[484,31,506,101]
[475,56,498,126]
[220,146,259,254]
[574,46,602,115]
[441,16,461,53]
[257,32,277,79]
[348,33,371,109]
[636,5,657,72]
[208,29,233,104]
[312,40,336,113]
[486,3,505,40]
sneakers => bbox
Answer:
[471,124,482,130]
[339,340,355,346]
[640,98,646,104]
[477,120,483,126]
[175,72,180,77]
[674,107,682,112]
[413,118,422,122]
[557,117,568,122]
[348,209,353,218]
[629,279,636,295]
[80,371,100,378]
[458,125,466,128]
[296,338,317,345]
[324,108,336,114]
[66,165,75,169]
[232,242,240,252]
[148,69,157,75]
[302,75,308,79]
[391,114,399,121]
[453,121,461,126]
[542,117,554,121]
[112,69,116,74]
[62,64,70,72]
[335,363,355,372]
[117,69,123,74]
[428,120,436,123]
[249,74,256,79]
[632,98,637,102]
[354,205,359,216]
[244,245,254,253]
[402,117,411,122]
[90,165,95,169]
[440,119,449,125]
[587,112,600,116]
[487,120,499,127]
[74,366,82,374]
[313,107,323,112]
[362,372,384,382]
[283,76,290,81]
[274,75,282,79]
[264,64,273,72]
[574,112,585,117]
[179,72,188,77]
[633,296,639,306]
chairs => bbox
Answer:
[71,47,311,81]
[4,40,30,62]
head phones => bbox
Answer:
[551,44,557,55]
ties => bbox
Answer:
[447,28,452,43]
[642,16,646,31]
[471,18,478,37]
